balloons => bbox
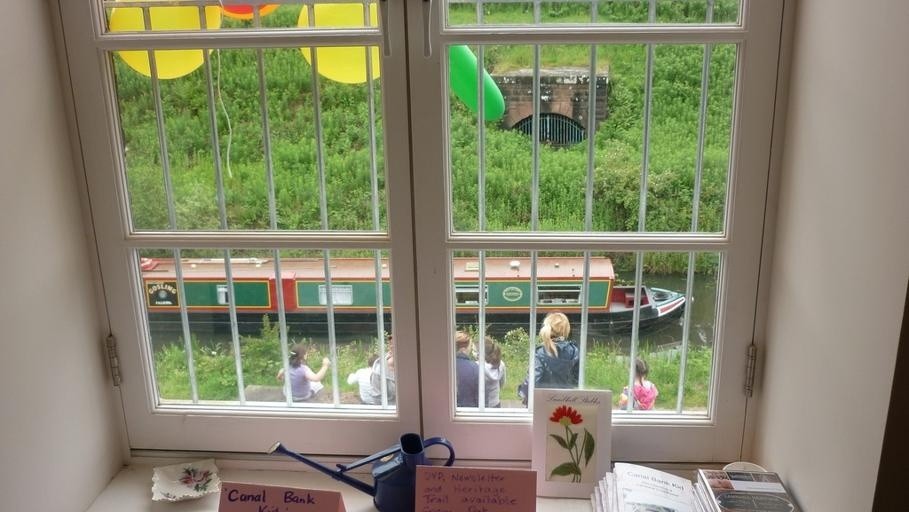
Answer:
[298,3,380,85]
[447,44,505,120]
[218,5,279,19]
[110,6,222,80]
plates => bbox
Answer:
[151,457,220,503]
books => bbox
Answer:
[589,462,801,512]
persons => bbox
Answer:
[517,312,579,408]
[347,354,379,405]
[618,360,659,410]
[277,345,331,402]
[369,336,396,405]
[472,337,507,407]
[455,330,479,407]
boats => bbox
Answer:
[141,256,686,334]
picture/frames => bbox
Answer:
[533,389,611,500]
